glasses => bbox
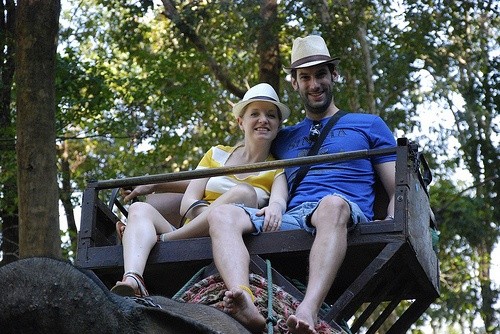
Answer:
[308,120,323,142]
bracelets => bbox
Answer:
[387,215,394,219]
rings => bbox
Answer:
[274,221,278,223]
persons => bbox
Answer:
[120,34,397,334]
[111,82,290,297]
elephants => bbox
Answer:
[0,250,354,334]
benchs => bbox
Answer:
[73,137,441,298]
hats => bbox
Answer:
[233,84,290,123]
[283,35,340,74]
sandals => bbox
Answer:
[116,221,127,239]
[109,272,149,297]
